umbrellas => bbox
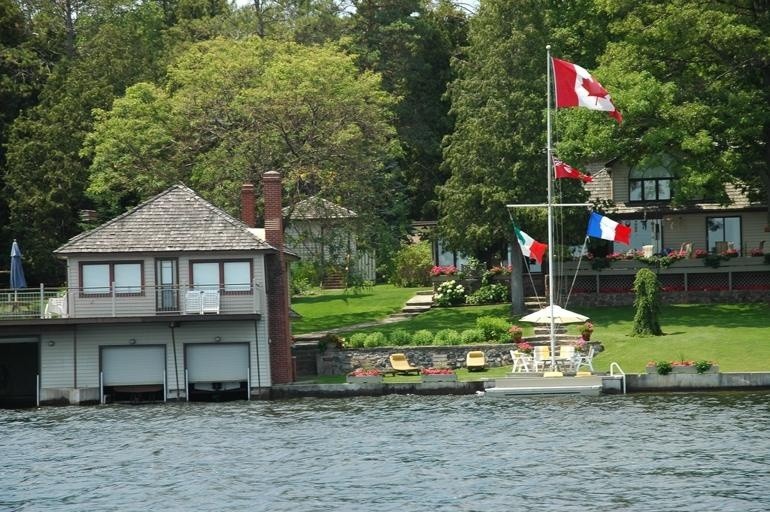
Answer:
[518,305,590,323]
[10,239,27,302]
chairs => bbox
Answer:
[390,353,420,377]
[510,344,596,374]
[183,290,221,314]
[43,292,68,318]
[466,351,486,371]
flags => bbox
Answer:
[586,212,632,244]
[512,219,547,265]
[552,158,592,182]
[551,56,622,124]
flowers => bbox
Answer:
[508,326,522,337]
[347,368,383,377]
[645,359,719,374]
[576,323,593,330]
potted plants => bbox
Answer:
[317,332,343,351]
[420,367,457,374]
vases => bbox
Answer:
[512,332,521,343]
[581,330,592,341]
